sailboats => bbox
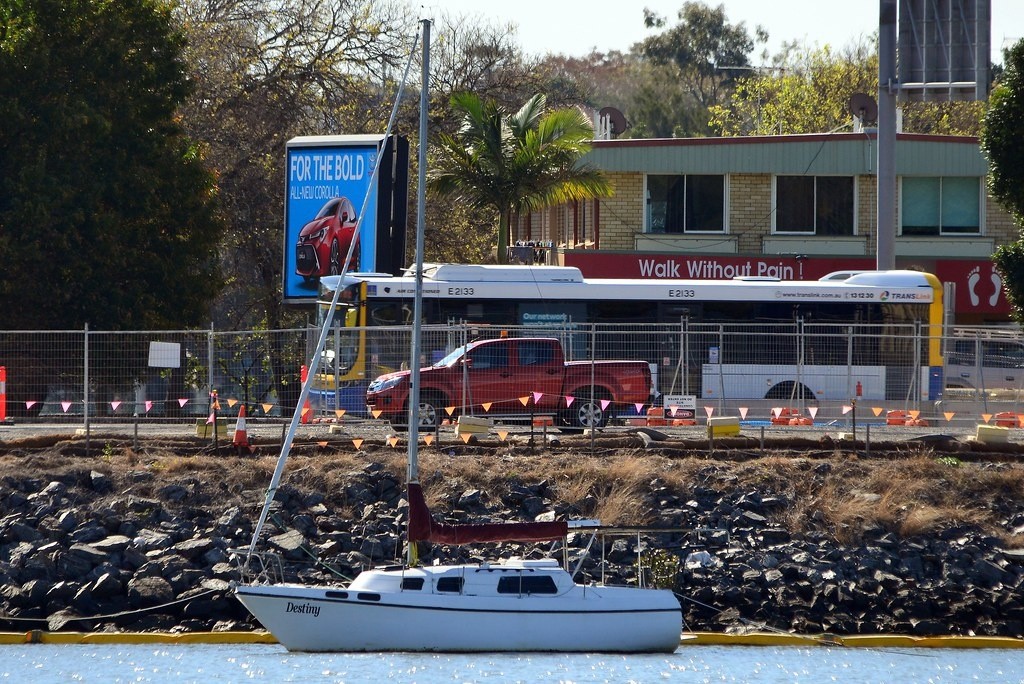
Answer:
[232,19,683,651]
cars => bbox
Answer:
[296,197,361,283]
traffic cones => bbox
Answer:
[0,367,14,425]
[233,406,249,447]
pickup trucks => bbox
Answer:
[366,330,652,438]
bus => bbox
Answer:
[309,264,948,421]
